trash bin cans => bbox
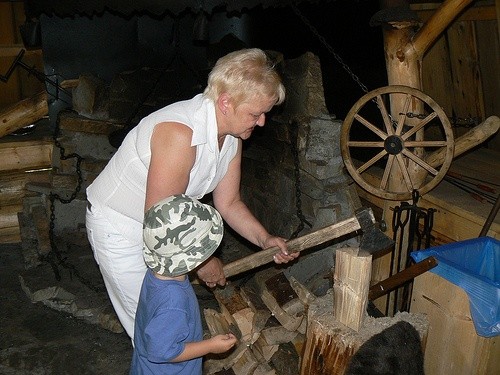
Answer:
[407,236,500,375]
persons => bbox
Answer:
[129,195,239,374]
[84,47,303,347]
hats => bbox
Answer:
[142,195,223,277]
